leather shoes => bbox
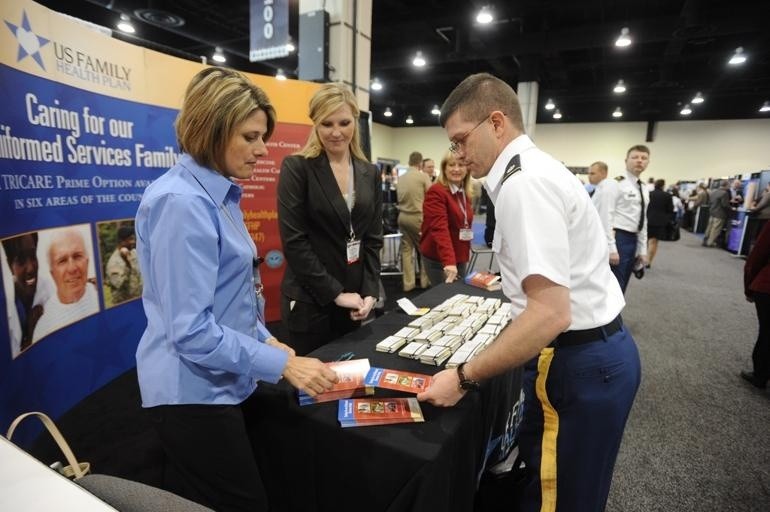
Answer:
[741,369,768,389]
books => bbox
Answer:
[367,363,432,397]
[298,358,375,404]
[464,270,502,292]
[337,397,424,426]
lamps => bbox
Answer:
[615,26,631,47]
[212,46,226,62]
[545,97,555,110]
[413,50,425,67]
[405,114,414,124]
[691,91,704,104]
[431,104,440,114]
[476,7,492,24]
[679,104,692,116]
[117,14,136,33]
[553,110,562,119]
[371,77,382,90]
[275,67,286,79]
[613,78,626,93]
[612,105,623,117]
[759,100,770,112]
[728,46,746,64]
[384,106,392,116]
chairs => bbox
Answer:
[380,234,405,271]
[465,243,496,277]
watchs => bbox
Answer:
[457,361,482,392]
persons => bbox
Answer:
[703,178,736,249]
[581,161,608,195]
[666,188,689,238]
[420,149,476,287]
[276,82,384,356]
[728,177,744,209]
[136,66,338,510]
[751,180,770,242]
[421,156,438,182]
[691,185,708,232]
[34,229,98,345]
[647,179,674,268]
[105,226,144,300]
[395,151,432,294]
[753,190,769,207]
[594,145,651,323]
[4,233,44,350]
[741,222,770,390]
[419,73,641,512]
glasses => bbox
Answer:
[449,114,507,153]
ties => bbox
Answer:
[637,179,645,231]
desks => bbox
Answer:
[255,272,531,512]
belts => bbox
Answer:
[614,228,637,237]
[557,314,623,344]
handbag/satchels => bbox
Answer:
[659,217,680,241]
